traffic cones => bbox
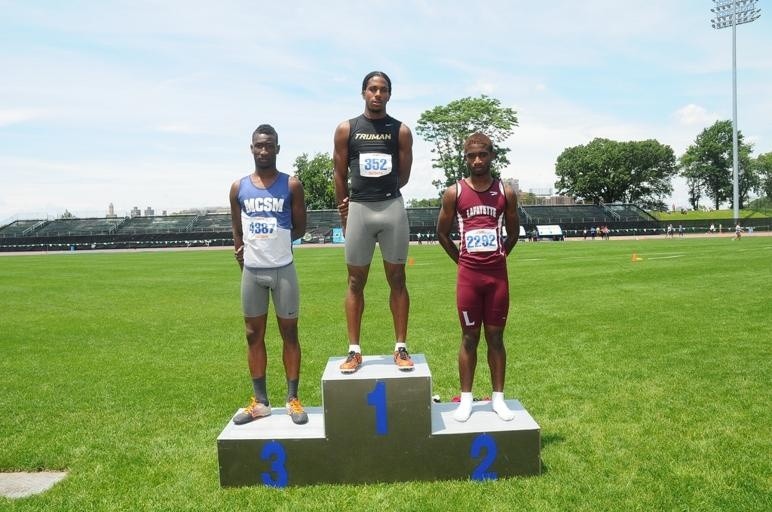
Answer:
[407,256,414,266]
[630,247,639,263]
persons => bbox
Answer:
[669,224,675,238]
[748,224,753,236]
[437,133,520,421]
[709,222,714,233]
[678,225,684,237]
[415,224,611,245]
[664,224,671,240]
[228,124,310,427]
[731,221,744,242]
[333,72,414,373]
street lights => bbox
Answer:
[706,0,763,227]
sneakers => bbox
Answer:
[285,396,309,425]
[232,397,273,425]
[392,346,414,371]
[338,350,363,374]
